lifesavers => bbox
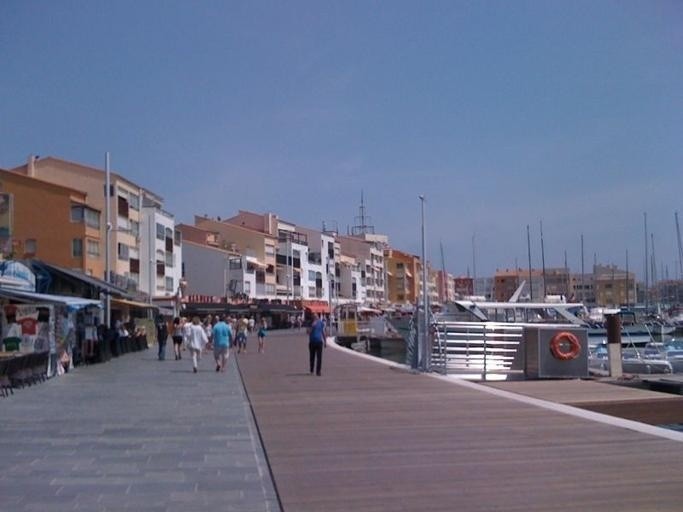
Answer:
[550,332,580,360]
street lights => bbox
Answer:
[418,194,430,372]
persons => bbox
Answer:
[306,311,326,377]
[116,313,146,337]
[155,314,168,360]
[171,312,268,374]
[290,312,304,332]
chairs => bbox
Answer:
[0,349,49,398]
